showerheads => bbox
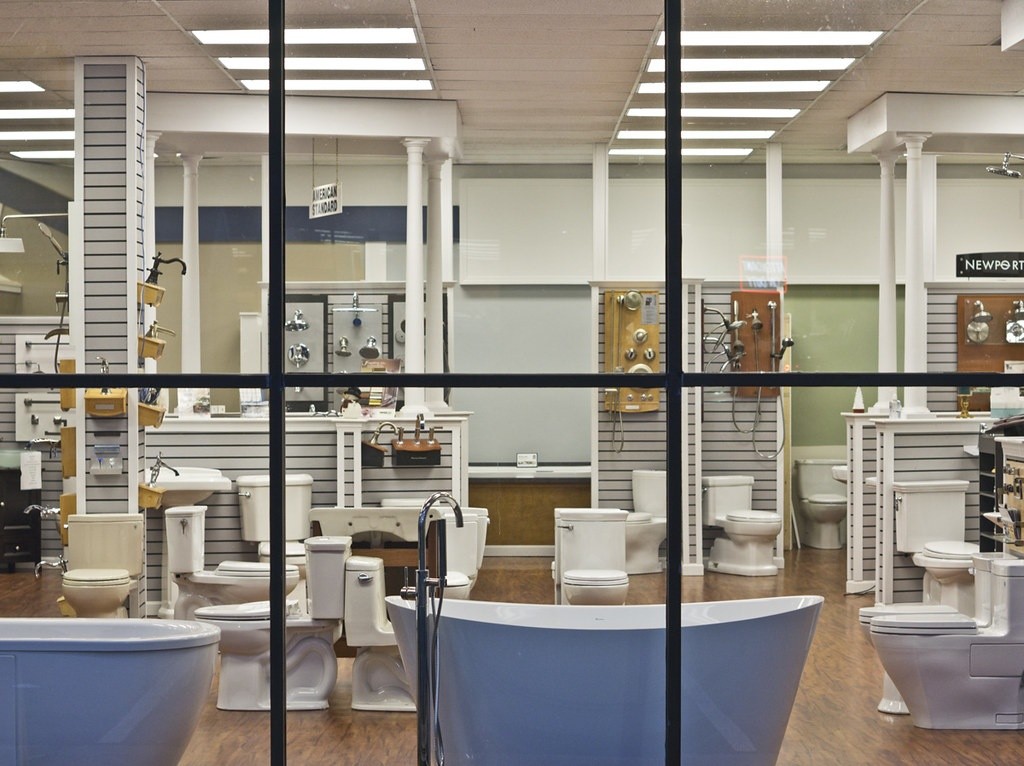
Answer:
[1004,297,1024,343]
[959,294,992,345]
[435,519,449,588]
[284,291,409,417]
[36,222,69,259]
[601,289,794,459]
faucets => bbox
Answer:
[138,253,189,489]
[23,504,61,520]
[43,327,69,342]
[370,423,398,448]
[412,414,427,444]
[35,557,69,582]
[416,491,463,572]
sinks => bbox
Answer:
[0,447,35,468]
[139,467,230,506]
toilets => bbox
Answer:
[61,474,491,713]
[546,458,1024,731]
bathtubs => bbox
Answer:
[0,616,221,765]
[383,592,821,763]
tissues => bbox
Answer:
[900,405,935,419]
[868,399,894,413]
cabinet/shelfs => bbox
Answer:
[0,467,47,572]
